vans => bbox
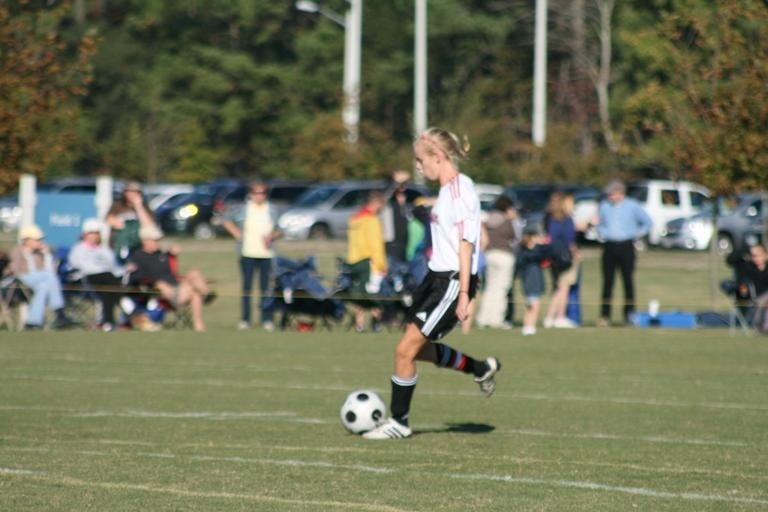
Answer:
[273,180,436,246]
[625,179,717,247]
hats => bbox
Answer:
[601,180,625,194]
[17,181,164,242]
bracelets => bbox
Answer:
[457,290,469,296]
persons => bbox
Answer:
[348,169,491,334]
[360,126,502,442]
[221,177,285,332]
[1,221,83,331]
[477,188,588,335]
[65,180,217,332]
[586,181,652,327]
[707,173,740,227]
[720,243,767,332]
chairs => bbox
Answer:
[335,256,394,333]
[274,255,332,332]
[717,274,762,337]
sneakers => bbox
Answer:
[361,415,414,441]
[473,354,500,400]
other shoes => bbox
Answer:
[476,315,637,336]
[238,315,404,333]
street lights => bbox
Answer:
[293,0,364,142]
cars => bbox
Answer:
[157,180,244,242]
[208,180,315,243]
[656,190,768,257]
[474,182,609,247]
[142,183,195,213]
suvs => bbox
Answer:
[0,174,145,232]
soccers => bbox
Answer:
[342,388,385,434]
